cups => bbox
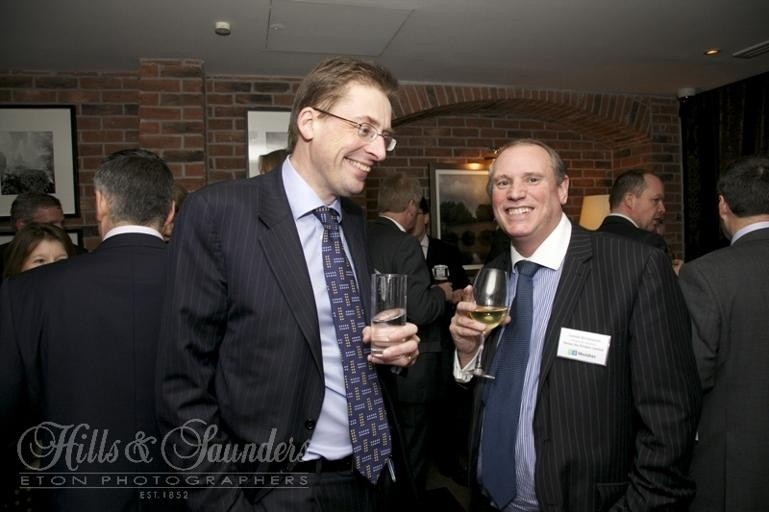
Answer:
[368,270,408,362]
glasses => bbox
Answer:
[310,105,398,152]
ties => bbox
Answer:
[480,261,544,508]
[312,207,392,484]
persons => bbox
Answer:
[446,138,702,510]
[146,57,424,510]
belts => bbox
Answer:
[285,453,355,478]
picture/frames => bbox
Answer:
[429,161,510,262]
[0,228,83,253]
[245,107,293,178]
[0,105,82,219]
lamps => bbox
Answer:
[579,194,611,231]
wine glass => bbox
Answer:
[467,266,510,380]
